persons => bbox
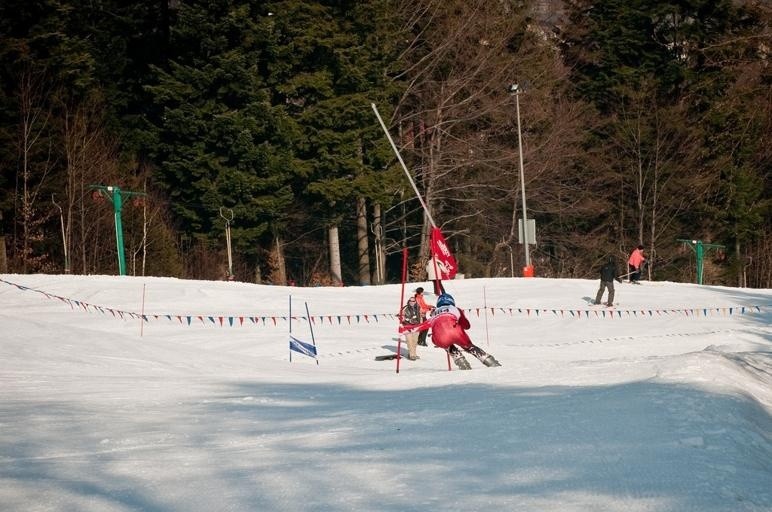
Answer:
[414,287,436,346]
[594,255,622,307]
[428,293,502,371]
[398,297,422,361]
[425,253,447,296]
[629,245,645,285]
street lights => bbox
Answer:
[508,82,539,277]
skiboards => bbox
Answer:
[587,300,620,310]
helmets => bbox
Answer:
[437,294,455,307]
